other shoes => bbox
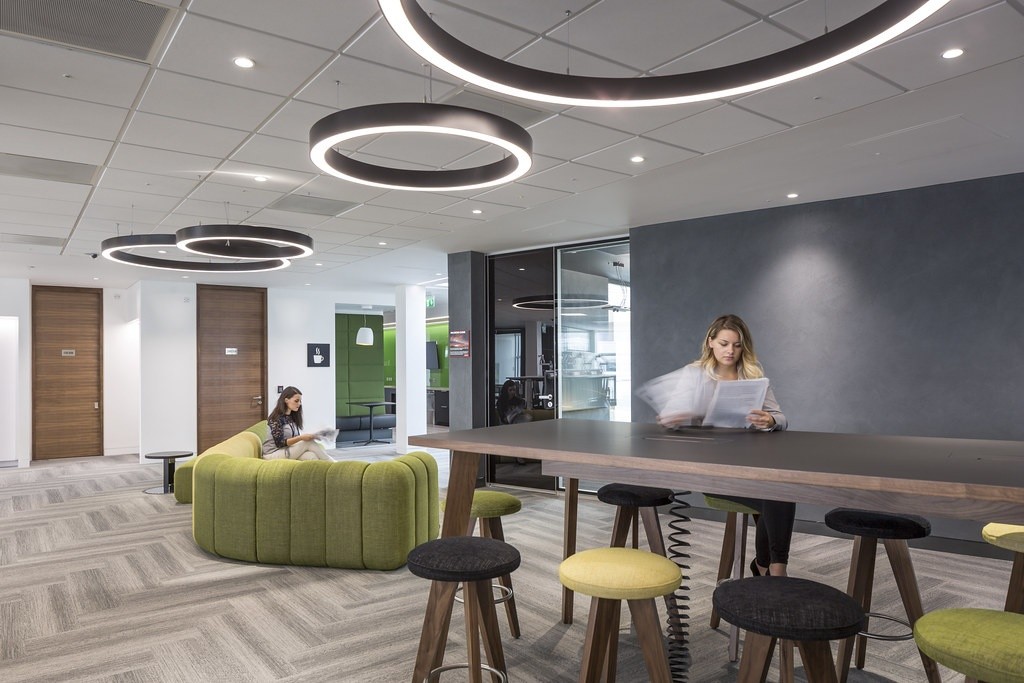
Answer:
[750,557,770,577]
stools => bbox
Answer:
[407,483,1024,683]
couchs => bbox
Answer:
[195,420,438,568]
[174,459,194,504]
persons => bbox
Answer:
[261,386,338,463]
[656,316,798,579]
[496,380,534,466]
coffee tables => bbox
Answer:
[145,450,193,494]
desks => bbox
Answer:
[346,401,396,446]
[383,385,449,426]
[409,418,1024,683]
[506,376,617,412]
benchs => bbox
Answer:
[336,413,396,441]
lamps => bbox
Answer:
[101,224,314,273]
[601,260,631,313]
[355,315,375,346]
[376,0,952,108]
[512,294,609,311]
[308,101,534,191]
